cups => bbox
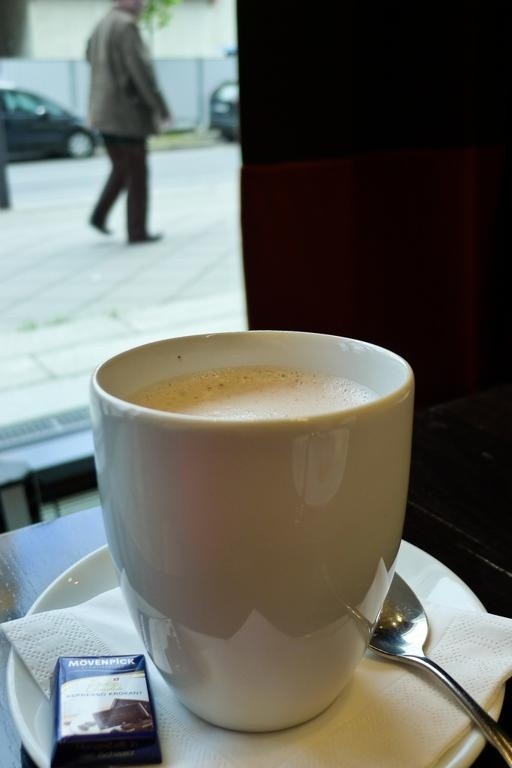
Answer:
[85,325,420,737]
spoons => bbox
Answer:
[366,569,512,767]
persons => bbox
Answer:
[86,0,169,243]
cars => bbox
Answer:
[0,86,99,164]
[208,79,240,144]
